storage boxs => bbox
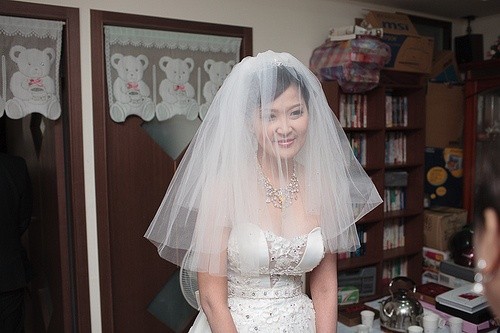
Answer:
[355,8,461,84]
[424,206,468,252]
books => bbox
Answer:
[337,224,367,259]
[384,188,404,212]
[339,91,367,128]
[383,260,407,279]
[345,134,367,167]
[435,283,489,314]
[384,130,407,164]
[385,96,407,127]
[382,218,404,250]
[477,92,500,130]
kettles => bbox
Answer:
[380,276,424,333]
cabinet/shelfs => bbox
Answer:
[464,58,500,230]
[306,62,429,328]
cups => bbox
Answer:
[361,311,375,331]
[423,316,438,333]
[408,326,423,333]
[449,318,462,333]
[358,325,369,333]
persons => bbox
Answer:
[143,49,383,333]
[471,131,500,324]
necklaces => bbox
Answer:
[256,154,298,211]
